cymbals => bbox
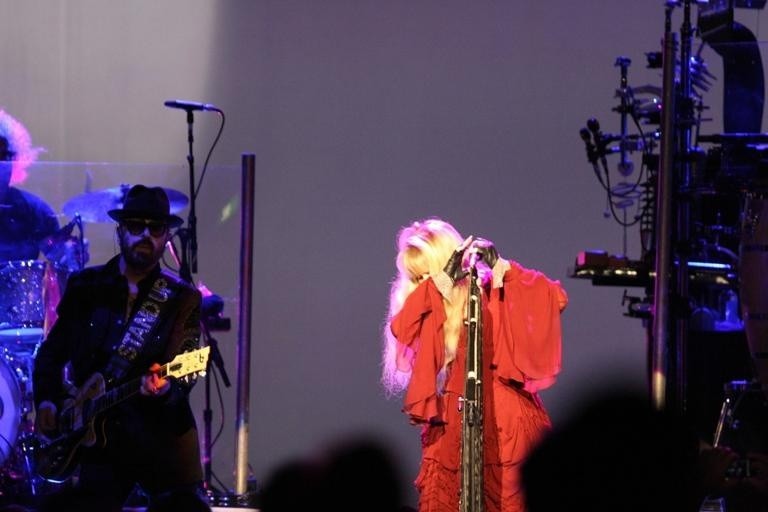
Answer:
[63,184,188,224]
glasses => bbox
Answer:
[126,220,165,238]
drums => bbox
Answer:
[0,345,32,462]
[0,261,66,345]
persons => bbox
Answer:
[0,135,61,258]
[258,439,416,511]
[31,184,208,511]
[696,443,766,510]
[520,387,705,510]
[145,490,211,512]
[379,217,569,511]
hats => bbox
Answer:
[107,184,184,227]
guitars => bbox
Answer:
[35,346,211,484]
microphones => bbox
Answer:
[468,237,485,267]
[164,99,207,112]
